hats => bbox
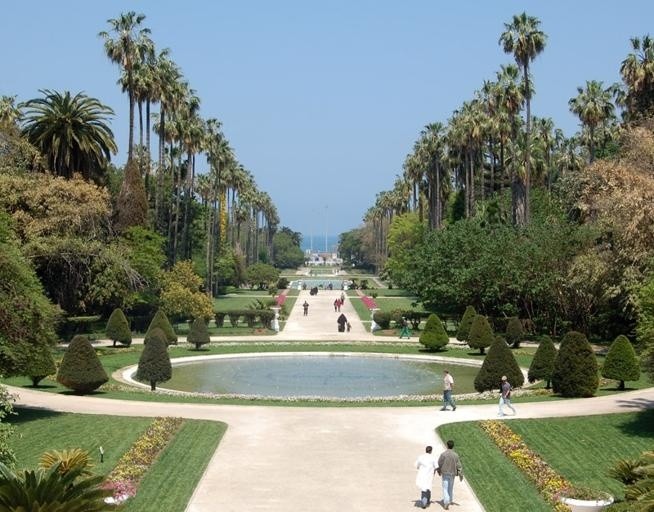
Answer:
[502,376,507,380]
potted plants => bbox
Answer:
[556,487,614,512]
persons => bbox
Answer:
[435,439,464,510]
[398,316,409,340]
[302,300,309,316]
[345,321,351,332]
[497,375,516,417]
[333,298,337,312]
[336,313,346,332]
[438,368,457,411]
[339,291,345,305]
[413,444,440,508]
[335,299,342,312]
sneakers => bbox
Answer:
[441,408,445,410]
[453,407,456,410]
